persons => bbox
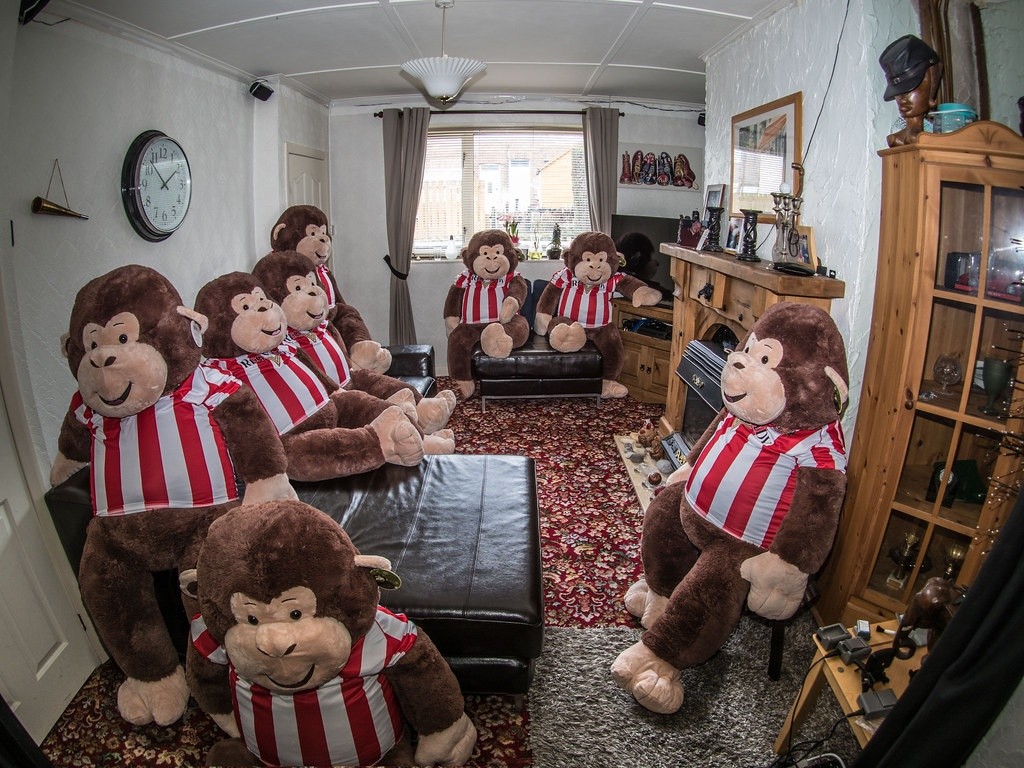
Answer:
[679,220,708,248]
[877,32,943,147]
[799,235,809,264]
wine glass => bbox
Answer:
[933,353,961,395]
[978,358,1012,414]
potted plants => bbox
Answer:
[508,222,519,243]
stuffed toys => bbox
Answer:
[537,233,664,398]
[609,303,849,715]
[178,499,477,768]
[194,204,457,481]
[444,228,529,399]
[51,263,297,726]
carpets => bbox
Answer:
[525,600,863,768]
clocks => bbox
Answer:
[121,130,192,243]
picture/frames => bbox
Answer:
[703,184,725,222]
[729,92,802,221]
[725,218,744,251]
[617,142,703,193]
[792,226,818,272]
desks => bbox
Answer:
[773,618,931,754]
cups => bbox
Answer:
[433,246,441,261]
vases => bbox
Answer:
[549,251,560,259]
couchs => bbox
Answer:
[471,278,603,412]
[45,342,546,695]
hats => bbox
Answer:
[880,35,935,103]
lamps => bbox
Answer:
[400,0,487,104]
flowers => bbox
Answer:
[547,223,563,253]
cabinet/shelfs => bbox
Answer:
[611,299,675,403]
[811,122,1024,630]
